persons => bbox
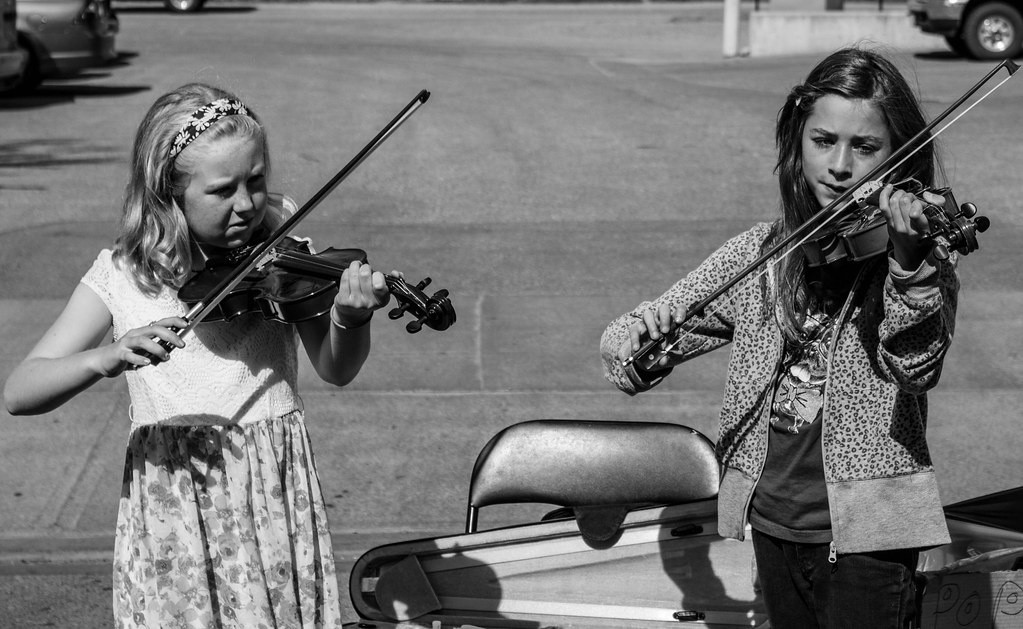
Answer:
[600,46,961,629]
[4,81,392,629]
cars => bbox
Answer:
[0,1,119,95]
[904,0,1023,62]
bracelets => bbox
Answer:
[331,305,373,330]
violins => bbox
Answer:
[800,180,991,269]
[177,229,457,334]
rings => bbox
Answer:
[149,321,156,326]
